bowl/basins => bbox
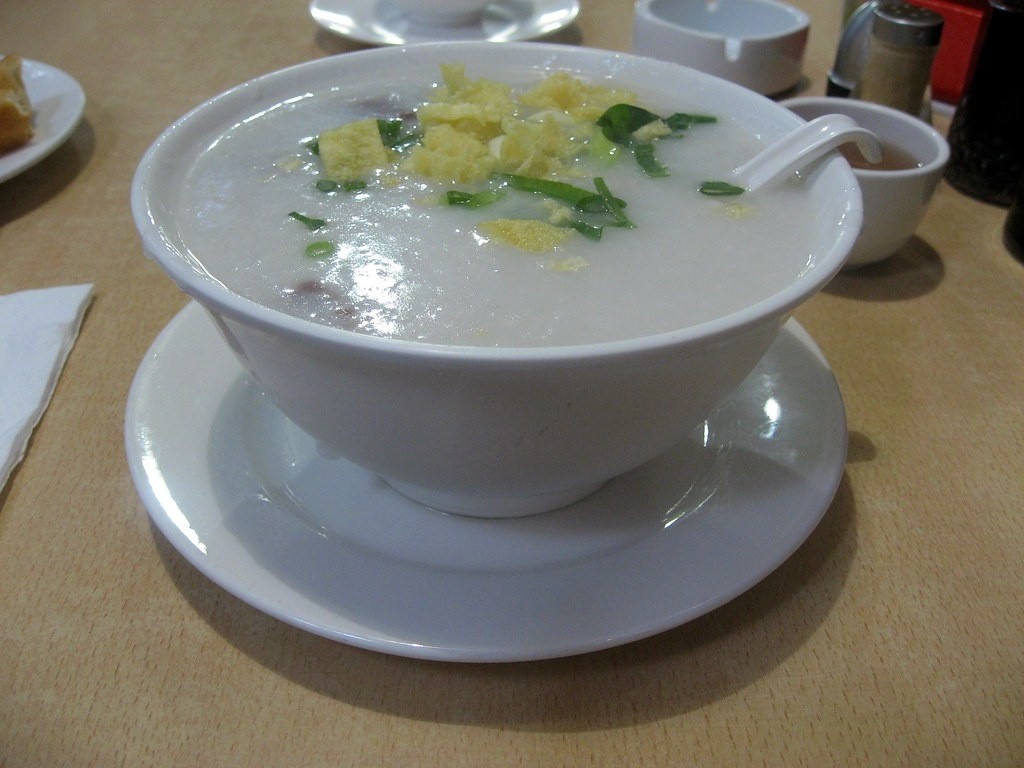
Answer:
[128,38,863,522]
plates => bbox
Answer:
[1,54,86,182]
[122,300,849,665]
[310,1,580,44]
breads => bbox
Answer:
[0,51,35,155]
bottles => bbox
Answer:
[860,6,945,117]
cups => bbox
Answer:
[398,0,487,27]
[780,97,951,275]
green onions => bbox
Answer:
[287,94,747,261]
